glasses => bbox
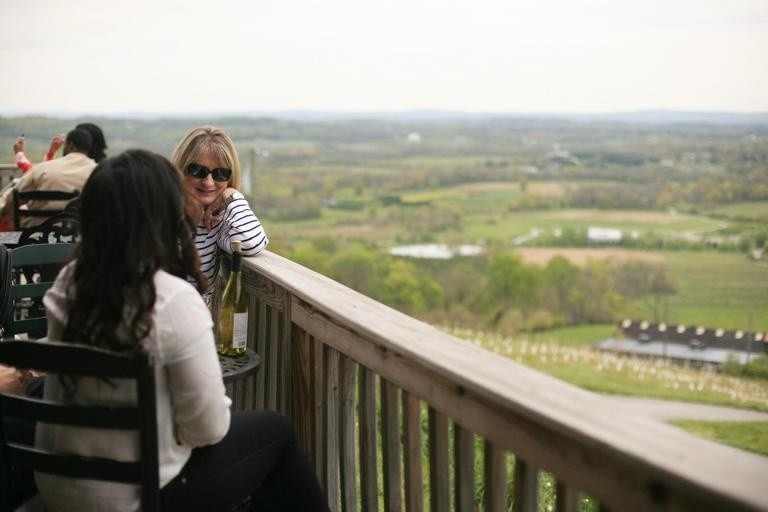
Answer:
[186,161,232,184]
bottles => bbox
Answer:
[219,241,248,358]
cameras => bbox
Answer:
[18,137,24,145]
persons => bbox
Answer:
[31,148,332,511]
[166,126,269,312]
[11,122,107,173]
[0,131,100,352]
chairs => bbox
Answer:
[2,186,162,512]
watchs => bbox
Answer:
[224,191,245,212]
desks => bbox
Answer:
[211,336,264,380]
